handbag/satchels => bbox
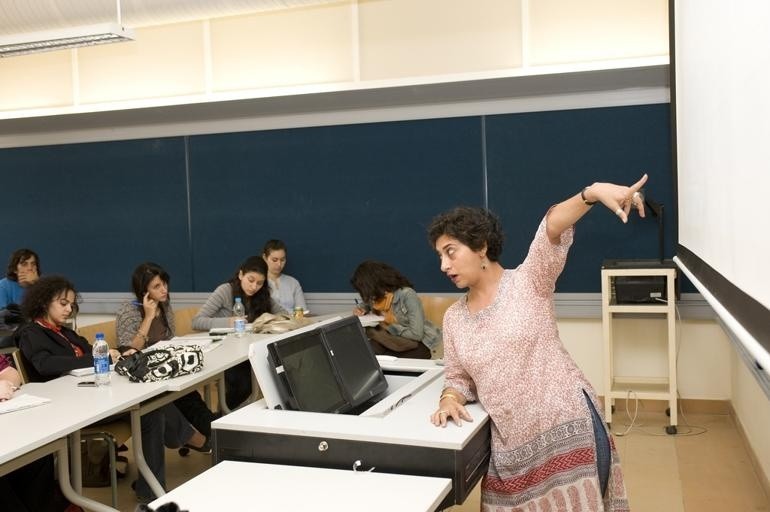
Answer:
[114,343,204,383]
[366,325,418,352]
[81,430,128,488]
[253,312,317,334]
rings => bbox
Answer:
[633,191,640,198]
[615,208,624,216]
[439,409,448,416]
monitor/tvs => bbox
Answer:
[267,327,352,414]
[318,315,388,407]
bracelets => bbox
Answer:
[135,328,150,343]
[439,390,462,402]
[579,183,597,209]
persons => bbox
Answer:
[0,351,83,511]
[262,238,310,314]
[114,260,226,440]
[425,173,661,510]
[19,276,213,501]
[0,247,41,312]
[348,257,443,360]
[190,255,293,409]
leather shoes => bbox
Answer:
[183,434,214,453]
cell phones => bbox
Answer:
[77,382,96,387]
[209,332,227,336]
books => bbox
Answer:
[146,320,258,352]
[66,364,113,377]
[0,389,53,415]
[358,311,386,327]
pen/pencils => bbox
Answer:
[131,300,143,305]
[354,298,361,310]
[11,387,21,390]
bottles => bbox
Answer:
[232,297,247,340]
[91,333,112,390]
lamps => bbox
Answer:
[0,0,135,61]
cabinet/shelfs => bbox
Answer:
[599,267,681,435]
